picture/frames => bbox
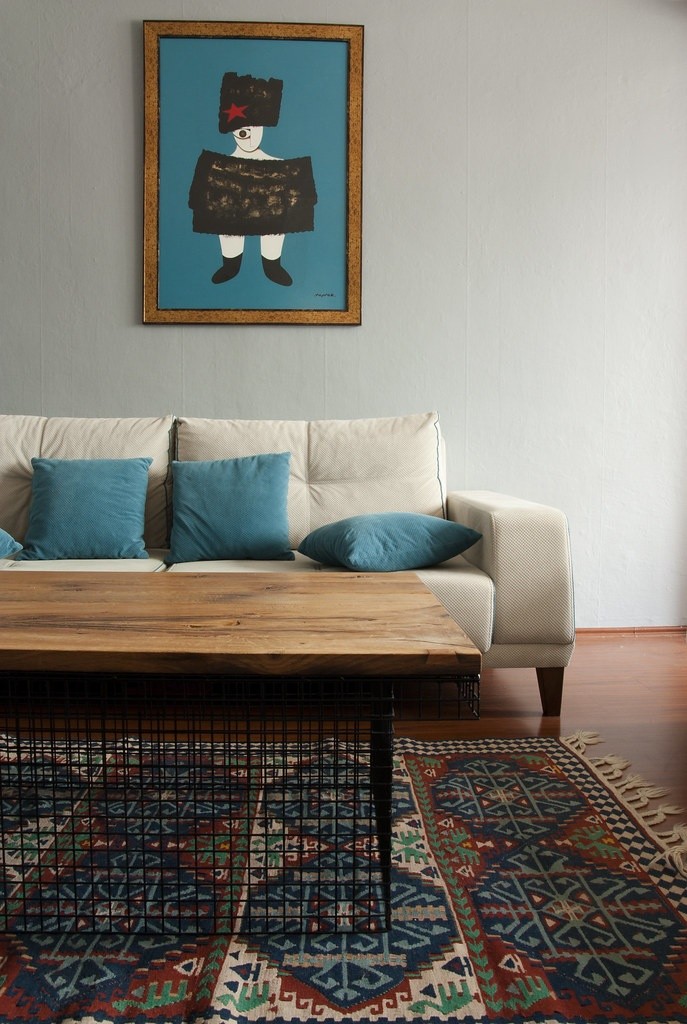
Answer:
[141,19,366,327]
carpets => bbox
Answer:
[0,728,687,1024]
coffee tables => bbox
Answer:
[0,570,482,937]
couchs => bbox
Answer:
[0,409,577,718]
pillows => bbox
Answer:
[13,457,153,560]
[163,450,295,564]
[297,512,483,573]
